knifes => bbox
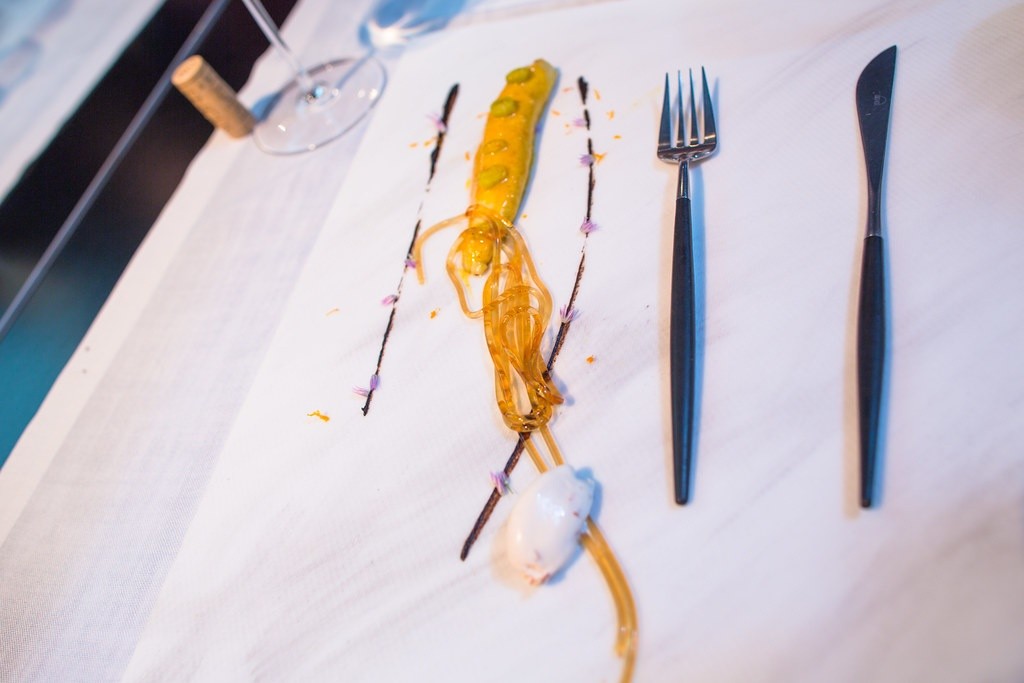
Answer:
[855,44,897,509]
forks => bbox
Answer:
[656,66,718,505]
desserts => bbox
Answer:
[462,58,559,272]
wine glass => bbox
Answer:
[242,0,387,155]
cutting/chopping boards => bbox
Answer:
[118,3,1023,679]
